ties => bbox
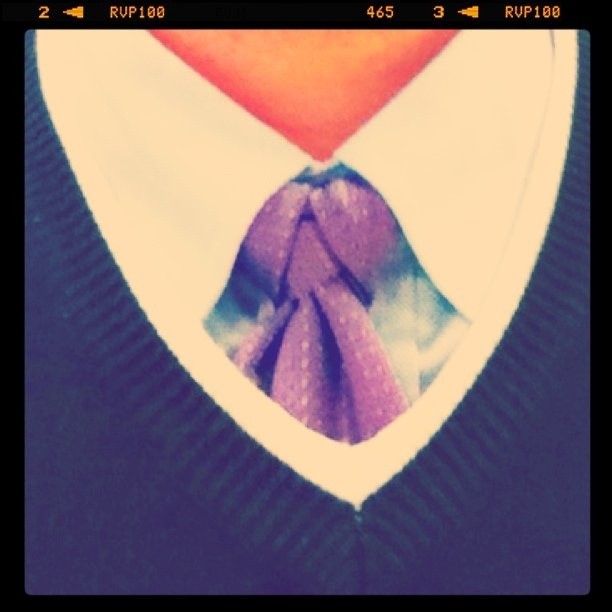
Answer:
[231,175,410,446]
